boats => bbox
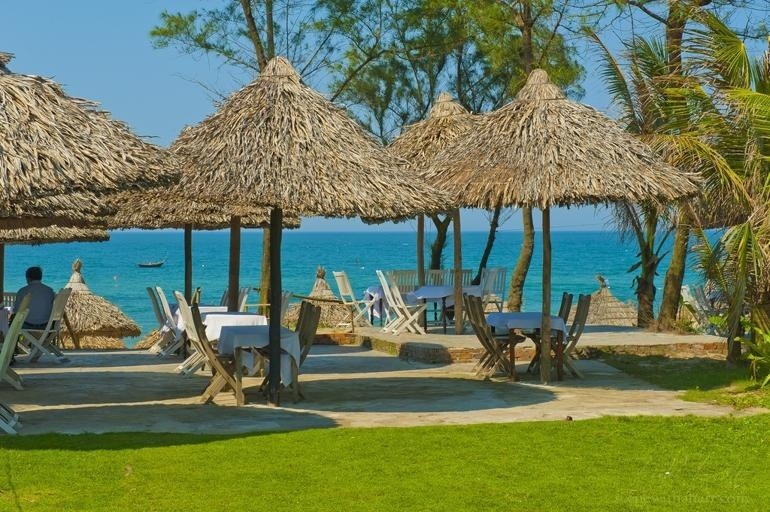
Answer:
[138,260,164,268]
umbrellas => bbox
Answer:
[415,68,699,380]
[358,90,488,334]
[162,57,451,401]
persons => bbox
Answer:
[8,265,56,366]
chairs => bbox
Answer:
[145,285,321,404]
[331,268,591,383]
[0,288,73,435]
